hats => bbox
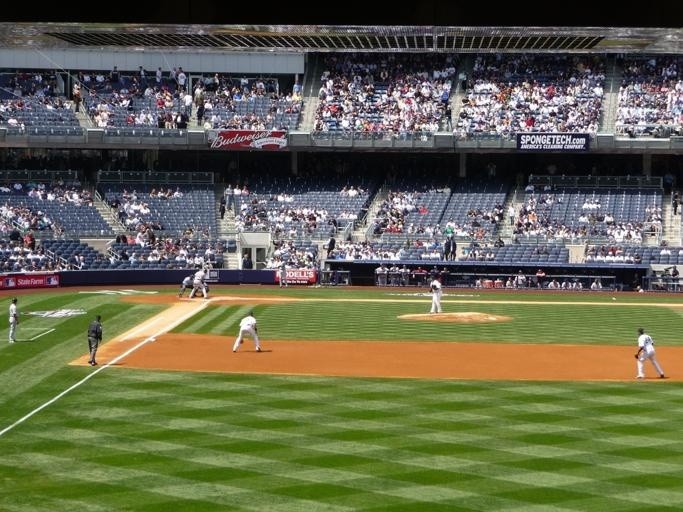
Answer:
[12,297,17,303]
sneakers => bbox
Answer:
[256,347,261,352]
[9,339,16,343]
[635,375,643,378]
[88,360,97,366]
[660,373,664,378]
[233,348,237,352]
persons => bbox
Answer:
[189,267,207,298]
[7,296,18,342]
[323,159,682,291]
[177,274,194,296]
[232,310,261,353]
[86,315,101,365]
[1,64,308,133]
[0,179,327,287]
[634,328,664,378]
[316,53,683,139]
[428,271,442,313]
